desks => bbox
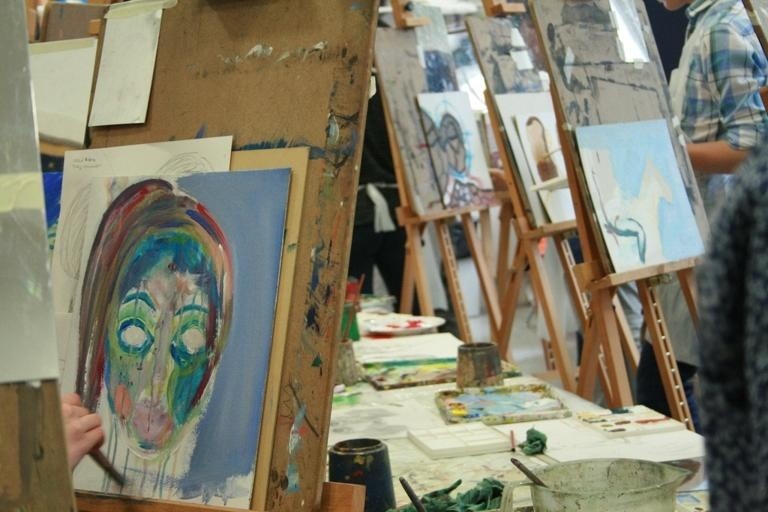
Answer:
[327,309,710,512]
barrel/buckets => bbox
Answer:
[522,459,691,512]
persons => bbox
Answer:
[632,0,768,437]
[345,68,422,319]
[57,388,106,477]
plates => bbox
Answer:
[369,314,448,337]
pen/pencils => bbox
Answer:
[89,449,126,487]
[342,272,367,342]
[511,430,515,452]
